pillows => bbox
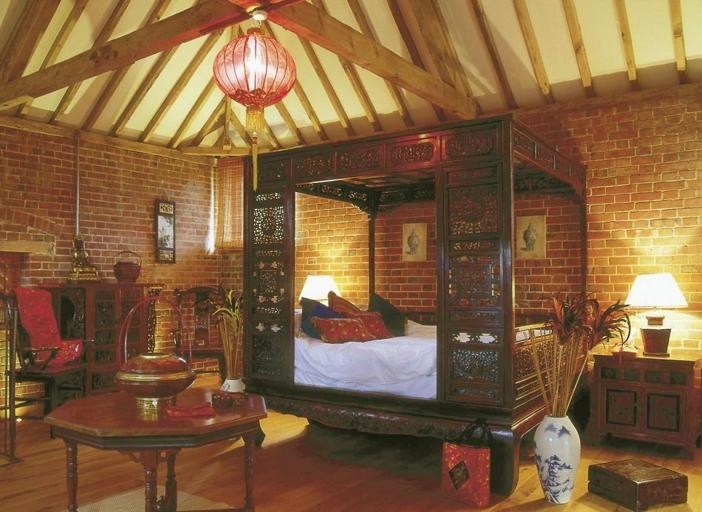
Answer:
[328,291,396,342]
[299,297,343,339]
[366,293,406,336]
[312,315,374,347]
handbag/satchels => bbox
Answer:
[440,441,492,508]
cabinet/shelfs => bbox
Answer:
[39,280,164,416]
[587,352,702,460]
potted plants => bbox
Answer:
[176,279,247,394]
[520,291,633,504]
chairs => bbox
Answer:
[176,286,229,381]
[2,284,87,442]
[177,279,231,393]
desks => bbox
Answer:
[43,386,266,511]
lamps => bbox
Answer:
[625,272,690,356]
[300,274,342,308]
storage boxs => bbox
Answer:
[587,459,689,510]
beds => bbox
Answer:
[241,113,602,500]
